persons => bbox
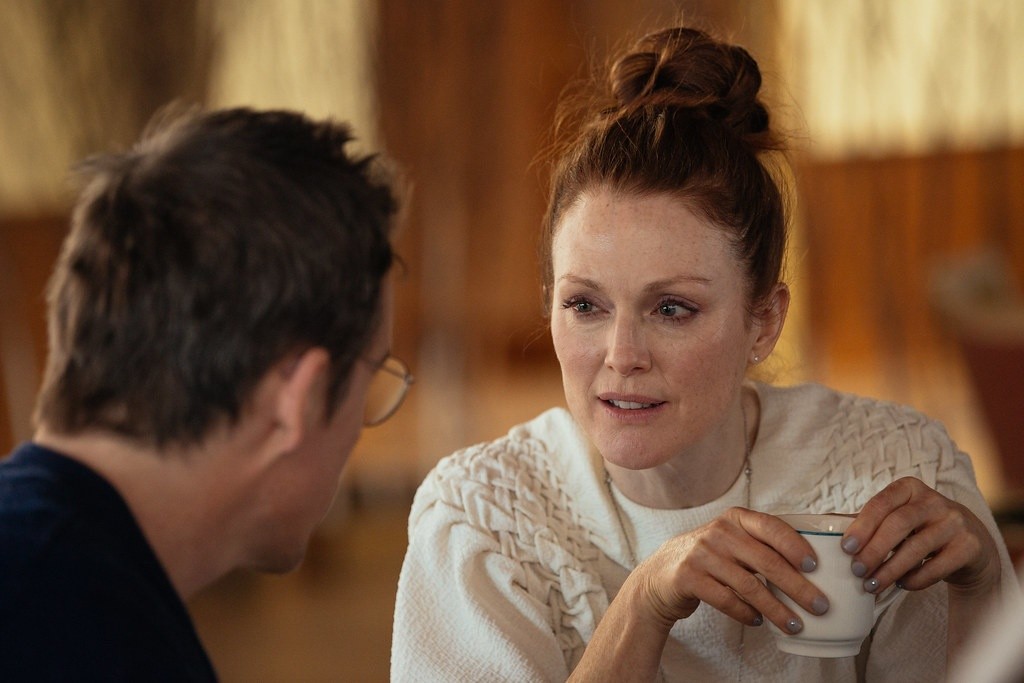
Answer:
[0,109,414,683]
[391,26,1024,683]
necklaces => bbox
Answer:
[604,399,752,683]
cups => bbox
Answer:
[756,511,911,659]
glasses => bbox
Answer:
[306,339,413,426]
